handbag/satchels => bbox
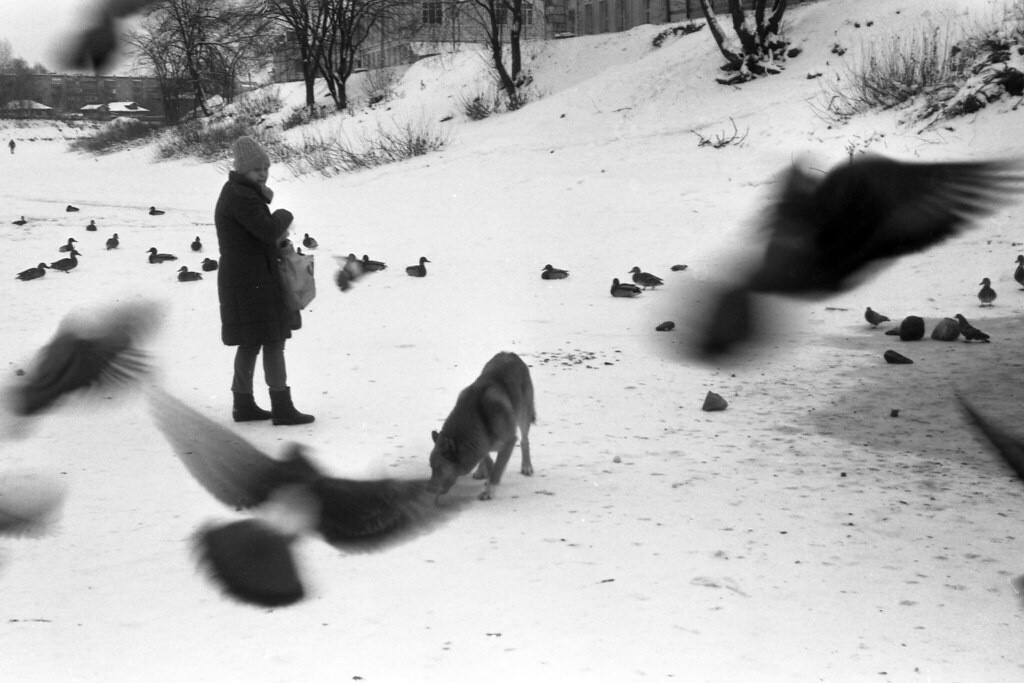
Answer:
[277,230,317,310]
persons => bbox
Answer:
[212,136,317,426]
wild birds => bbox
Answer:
[610,278,642,297]
[541,264,570,279]
[177,266,203,282]
[145,247,178,264]
[15,238,81,281]
[12,216,27,225]
[201,258,218,271]
[148,206,165,215]
[406,256,431,278]
[86,219,98,231]
[697,153,1024,363]
[332,253,387,291]
[190,236,202,251]
[65,205,80,212]
[628,266,664,290]
[105,233,119,250]
[295,232,317,257]
[0,298,488,609]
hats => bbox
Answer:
[232,136,271,175]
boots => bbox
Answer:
[268,386,315,426]
[233,390,272,423]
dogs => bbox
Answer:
[424,351,536,503]
